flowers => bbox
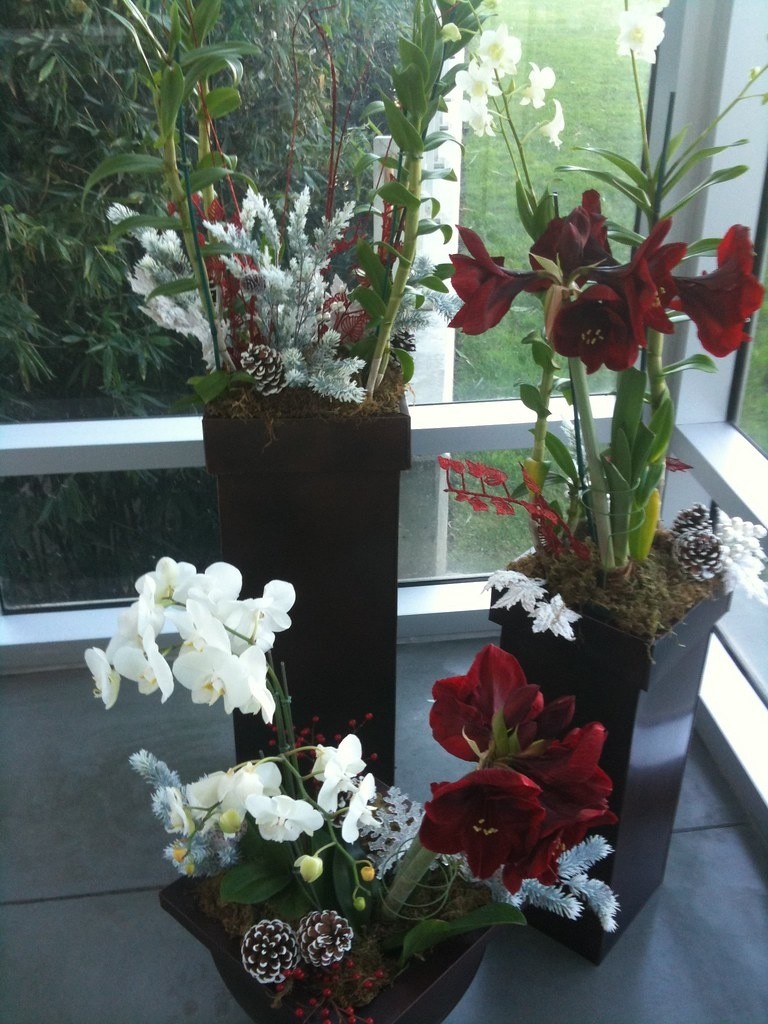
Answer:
[78,0,499,409]
[83,556,620,1024]
[436,0,768,642]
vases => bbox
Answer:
[200,393,413,796]
[487,545,733,968]
[158,868,492,1024]
